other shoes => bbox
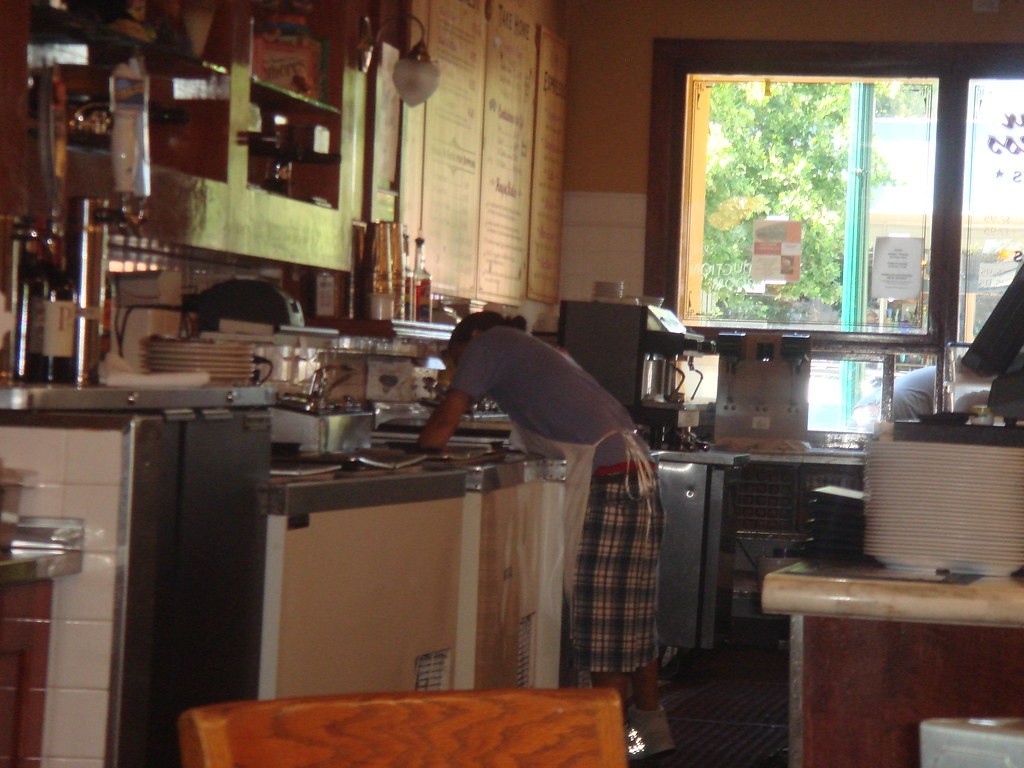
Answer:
[626,702,676,762]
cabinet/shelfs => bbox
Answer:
[0,0,375,273]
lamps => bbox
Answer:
[357,13,442,110]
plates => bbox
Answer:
[592,281,664,307]
[918,413,969,424]
[139,341,254,387]
[862,439,1024,578]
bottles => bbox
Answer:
[403,234,413,320]
[27,205,76,383]
[413,237,432,322]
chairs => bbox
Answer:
[178,684,630,768]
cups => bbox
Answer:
[264,162,292,196]
[346,220,405,321]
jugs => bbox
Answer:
[0,214,50,378]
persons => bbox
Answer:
[894,356,980,421]
[419,307,676,765]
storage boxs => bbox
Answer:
[366,358,413,401]
[413,367,446,400]
[269,405,373,454]
[321,355,365,403]
